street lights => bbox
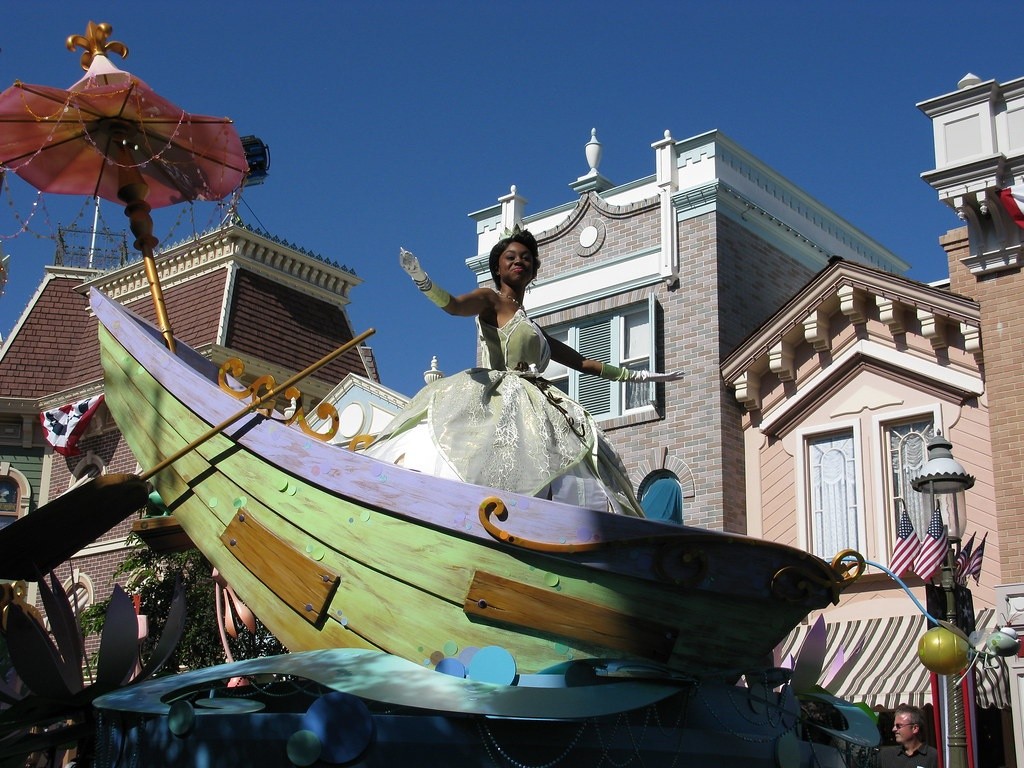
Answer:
[911,429,979,767]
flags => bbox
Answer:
[913,507,950,583]
[887,506,921,580]
[956,536,985,588]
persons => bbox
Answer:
[873,706,940,768]
[363,224,688,521]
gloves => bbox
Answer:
[599,362,685,382]
[400,247,449,307]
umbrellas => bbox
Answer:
[0,22,250,355]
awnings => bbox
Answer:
[779,608,1011,709]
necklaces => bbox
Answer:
[497,291,525,311]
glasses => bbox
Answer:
[893,723,916,729]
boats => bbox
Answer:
[85,283,845,724]
